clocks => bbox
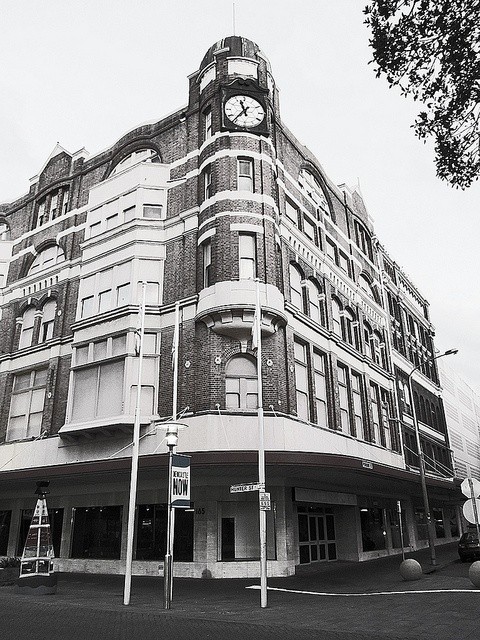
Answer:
[223,93,266,129]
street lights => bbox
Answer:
[155,422,189,609]
[408,348,458,565]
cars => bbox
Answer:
[457,532,480,561]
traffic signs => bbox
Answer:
[229,482,262,494]
